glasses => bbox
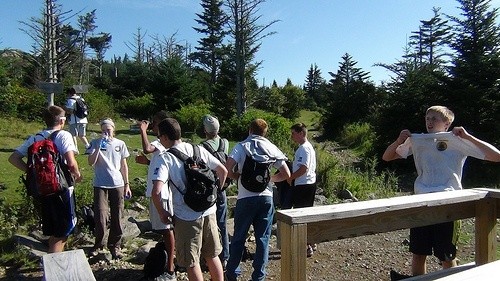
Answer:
[60,116,67,121]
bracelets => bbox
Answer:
[124,182,129,186]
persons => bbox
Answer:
[8,106,82,254]
[62,88,91,156]
[140,111,174,274]
[288,123,317,257]
[198,117,229,271]
[274,159,294,210]
[88,118,132,259]
[152,118,228,281]
[383,106,500,277]
[224,119,291,281]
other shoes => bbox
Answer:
[311,243,317,251]
[223,259,238,281]
[112,248,124,261]
[89,248,101,257]
[307,247,313,257]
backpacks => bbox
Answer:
[21,129,75,202]
[69,96,89,127]
[164,142,218,212]
[142,248,168,279]
[200,140,232,193]
[237,155,273,193]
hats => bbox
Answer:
[203,114,220,135]
[100,117,116,128]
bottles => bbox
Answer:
[266,180,278,192]
[100,133,109,148]
[130,123,154,132]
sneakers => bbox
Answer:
[154,272,177,281]
